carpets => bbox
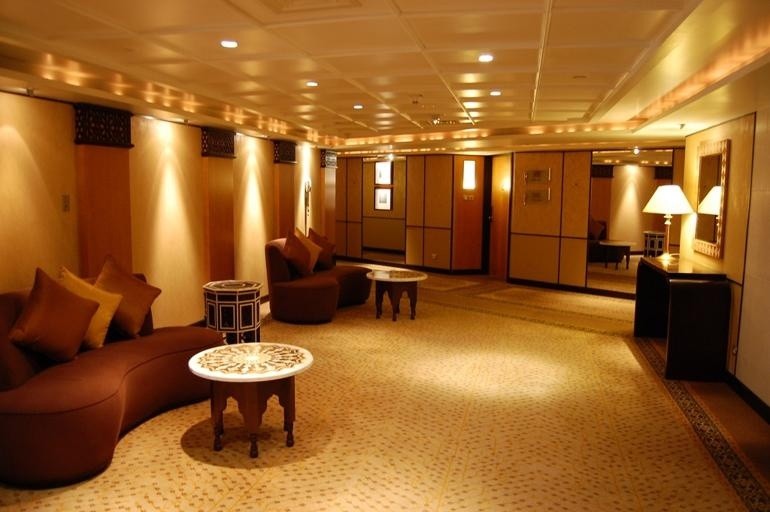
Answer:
[0,276,769,512]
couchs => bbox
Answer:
[265,237,372,325]
[0,272,226,489]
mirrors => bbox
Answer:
[693,139,731,259]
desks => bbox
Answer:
[635,257,730,382]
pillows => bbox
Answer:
[8,256,161,363]
[282,226,337,276]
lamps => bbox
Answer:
[697,186,722,215]
[642,185,694,258]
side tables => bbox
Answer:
[642,231,666,257]
[202,279,263,344]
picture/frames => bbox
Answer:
[373,161,393,211]
